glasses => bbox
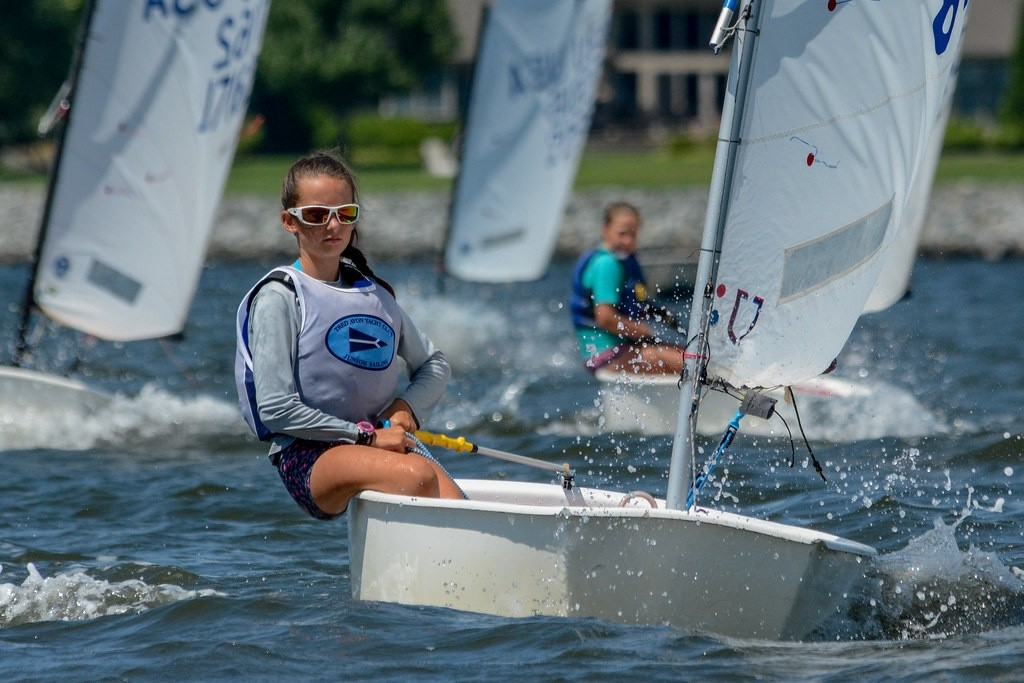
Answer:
[287,204,360,226]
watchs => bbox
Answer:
[357,421,375,446]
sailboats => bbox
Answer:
[343,0,976,643]
[399,0,614,374]
[1,0,271,458]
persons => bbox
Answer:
[232,153,474,520]
[568,199,687,374]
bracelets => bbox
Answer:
[367,433,374,448]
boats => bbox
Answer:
[583,356,879,446]
